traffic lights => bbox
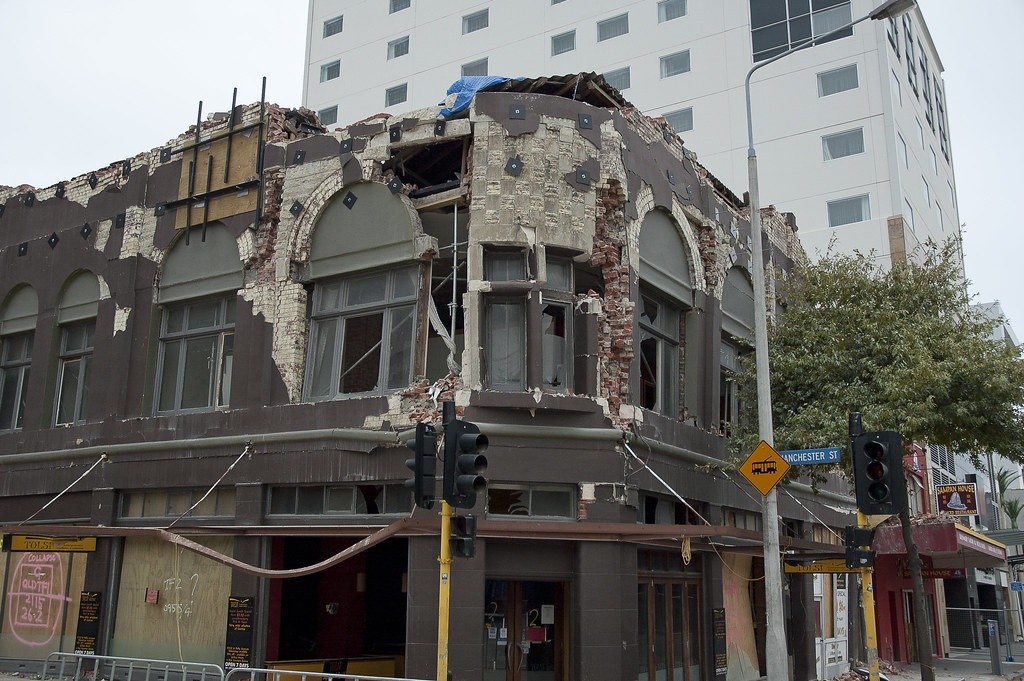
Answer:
[404,422,438,510]
[854,431,905,515]
[846,527,875,571]
[448,516,477,559]
[442,419,489,509]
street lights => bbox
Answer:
[743,0,920,681]
